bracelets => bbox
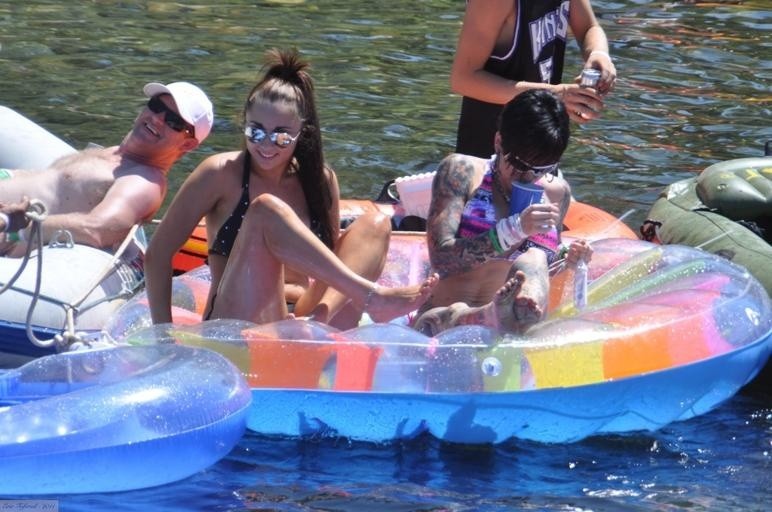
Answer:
[487,216,526,253]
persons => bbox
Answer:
[447,1,618,158]
[0,80,214,258]
[415,88,593,337]
[0,194,31,235]
[145,44,436,334]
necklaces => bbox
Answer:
[492,163,511,203]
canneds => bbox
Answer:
[580,69,603,114]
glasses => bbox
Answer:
[147,95,194,137]
[499,144,559,178]
[243,124,302,149]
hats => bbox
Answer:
[142,80,214,146]
